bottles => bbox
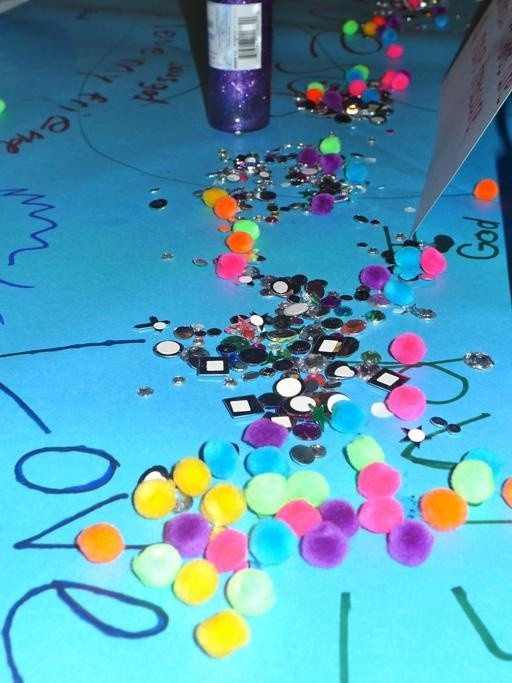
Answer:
[203,0,275,132]
